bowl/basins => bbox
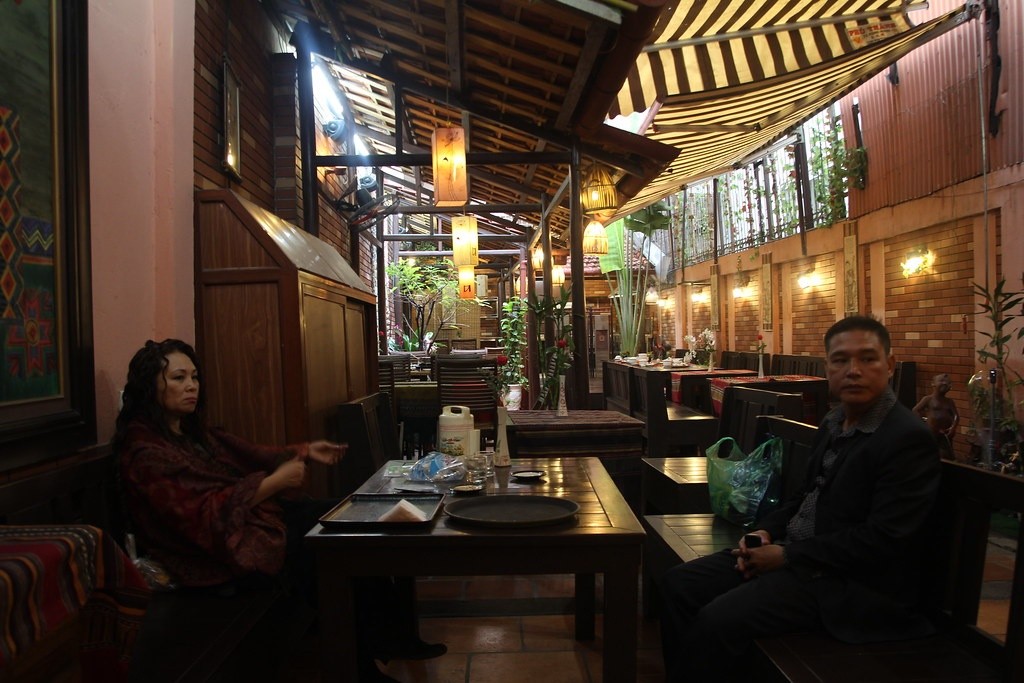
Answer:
[624,356,637,363]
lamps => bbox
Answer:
[532,155,929,305]
[322,17,488,300]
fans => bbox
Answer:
[332,190,401,233]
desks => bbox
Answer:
[506,409,646,513]
[709,374,830,422]
[670,369,758,406]
[0,524,151,683]
[608,359,724,400]
[392,380,528,440]
[302,457,647,683]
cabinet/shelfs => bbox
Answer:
[195,187,378,464]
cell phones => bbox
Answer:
[745,533,762,548]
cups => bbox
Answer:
[474,450,496,477]
[663,360,672,368]
[465,455,487,484]
[615,354,647,367]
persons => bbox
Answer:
[650,315,940,683]
[913,373,959,459]
[653,331,671,358]
[115,338,448,683]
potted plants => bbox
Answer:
[492,298,529,411]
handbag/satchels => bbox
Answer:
[704,436,784,531]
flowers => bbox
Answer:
[552,341,568,374]
[684,335,698,360]
[477,354,515,406]
[756,334,767,355]
[698,327,716,355]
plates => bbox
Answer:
[663,358,683,361]
[449,484,486,494]
[511,471,546,479]
[673,364,688,367]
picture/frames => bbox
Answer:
[221,53,243,183]
[0,0,101,481]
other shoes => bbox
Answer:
[348,668,401,682]
[375,637,449,666]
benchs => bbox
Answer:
[1,351,1024,683]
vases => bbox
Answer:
[556,374,569,417]
[708,353,715,373]
[759,355,765,379]
[494,407,511,468]
